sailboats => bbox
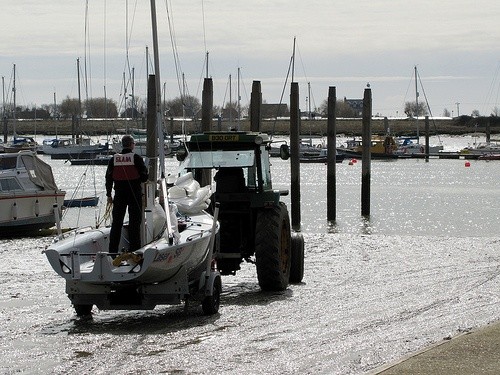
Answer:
[0,0,499,163]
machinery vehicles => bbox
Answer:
[172,134,304,292]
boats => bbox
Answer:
[0,157,65,241]
[64,193,100,213]
[44,174,220,282]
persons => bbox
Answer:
[105,135,148,260]
